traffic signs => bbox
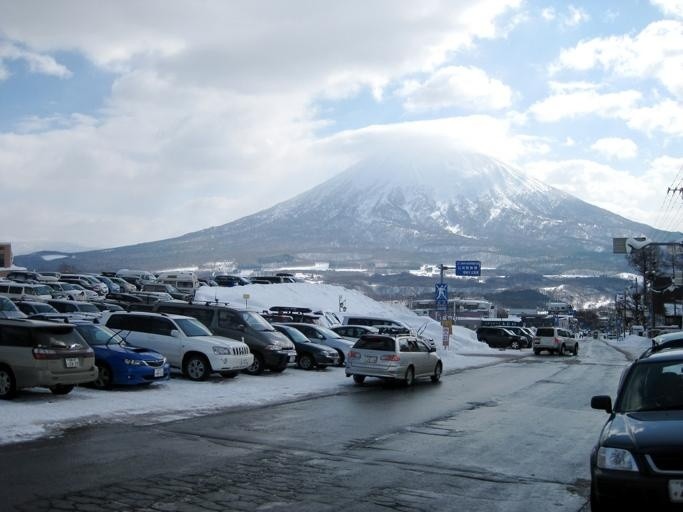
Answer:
[455,260,481,276]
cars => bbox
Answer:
[589,347,682,512]
[584,327,617,339]
[616,331,682,402]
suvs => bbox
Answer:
[628,325,644,337]
[475,324,580,356]
